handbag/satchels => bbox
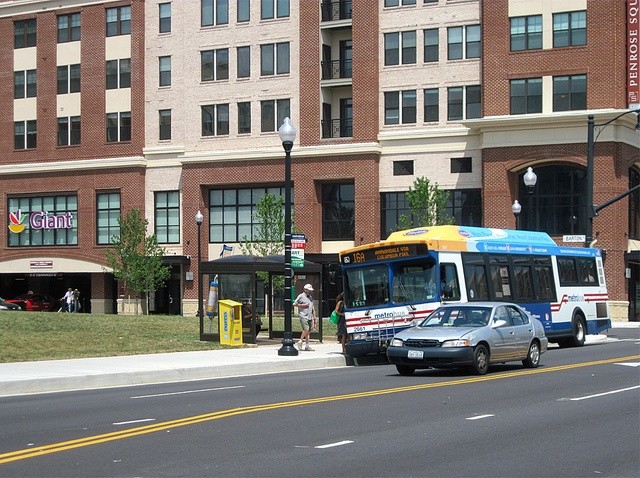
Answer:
[329,305,344,325]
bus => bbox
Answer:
[336,223,612,361]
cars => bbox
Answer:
[387,302,550,375]
[4,293,59,312]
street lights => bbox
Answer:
[277,117,299,357]
[523,166,537,230]
[195,210,204,342]
[512,200,521,230]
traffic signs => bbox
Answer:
[291,233,305,268]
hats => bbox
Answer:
[304,284,315,291]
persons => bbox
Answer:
[292,284,317,351]
[329,292,347,354]
[60,288,81,313]
